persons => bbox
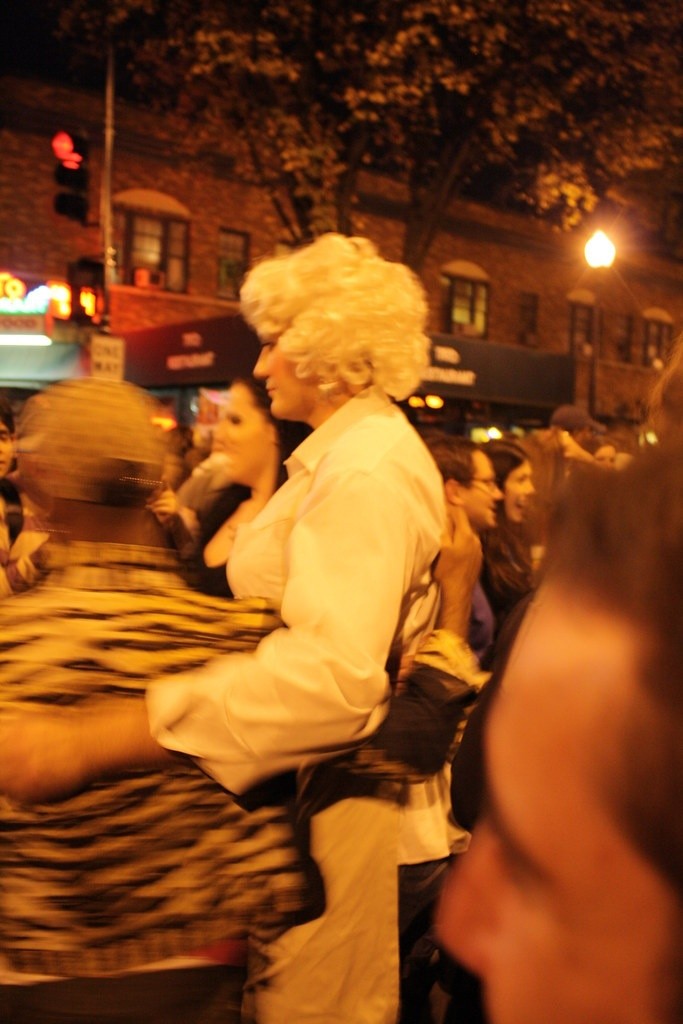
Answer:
[0,232,683,1024]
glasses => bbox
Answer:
[469,475,499,492]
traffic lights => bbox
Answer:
[51,131,90,191]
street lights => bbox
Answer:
[585,228,621,420]
